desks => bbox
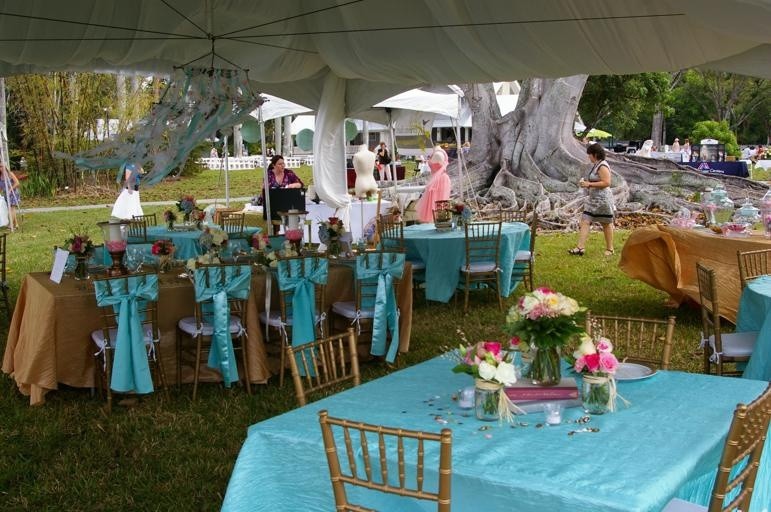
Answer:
[0,249,414,407]
[279,198,392,245]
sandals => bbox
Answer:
[568,245,585,256]
[603,248,615,256]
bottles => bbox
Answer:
[359,237,365,253]
[285,240,292,256]
[451,215,457,232]
[463,205,471,230]
[578,176,584,193]
[703,183,771,240]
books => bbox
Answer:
[504,378,583,414]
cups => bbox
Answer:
[458,388,474,408]
[376,243,382,250]
[542,399,569,426]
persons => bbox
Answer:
[210,142,274,157]
[652,137,764,162]
[568,143,614,256]
[415,145,451,224]
[352,144,378,198]
[110,163,145,219]
[378,143,392,181]
[0,163,20,230]
[262,154,304,188]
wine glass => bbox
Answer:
[124,247,146,273]
[229,241,241,264]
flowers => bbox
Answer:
[55,222,96,260]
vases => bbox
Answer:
[75,257,90,280]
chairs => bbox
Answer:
[0,233,13,323]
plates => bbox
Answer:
[613,359,657,380]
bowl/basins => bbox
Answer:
[305,243,320,250]
